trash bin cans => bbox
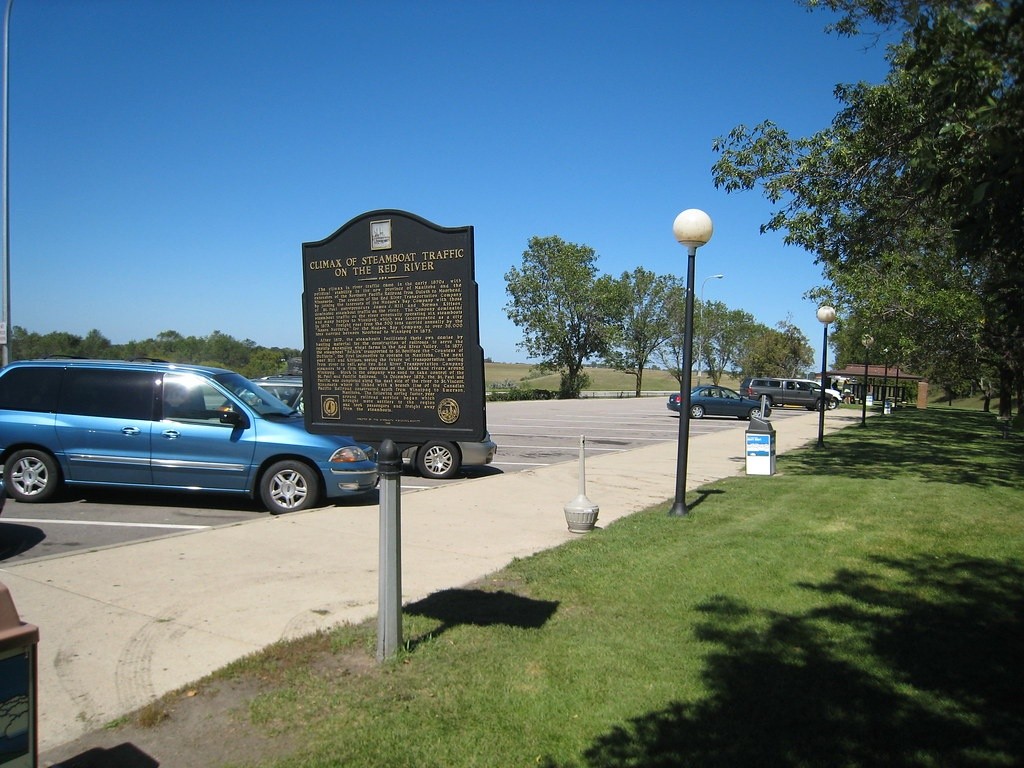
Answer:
[884,398,892,414]
[1,583,41,767]
[745,416,776,475]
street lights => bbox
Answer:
[662,207,714,517]
[695,275,724,386]
[816,306,837,450]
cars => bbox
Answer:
[666,385,772,420]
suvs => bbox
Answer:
[739,377,832,414]
[218,373,499,482]
[0,355,380,518]
[791,379,843,410]
